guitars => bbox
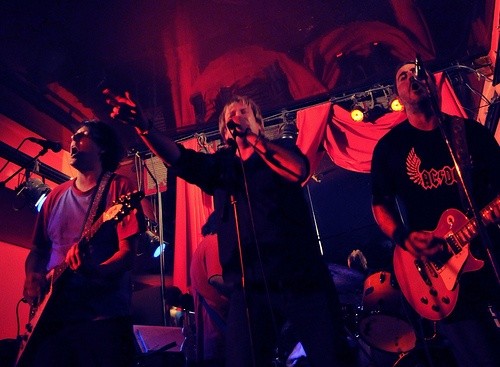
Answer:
[14,189,146,367]
[392,193,500,320]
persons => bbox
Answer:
[370,63,500,367]
[103,90,355,367]
[23,120,144,367]
[190,210,238,367]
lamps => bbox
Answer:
[139,231,169,258]
[382,87,405,112]
[351,93,374,122]
[280,114,295,140]
[12,169,51,214]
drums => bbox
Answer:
[356,272,419,352]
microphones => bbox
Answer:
[415,55,425,80]
[29,137,62,152]
